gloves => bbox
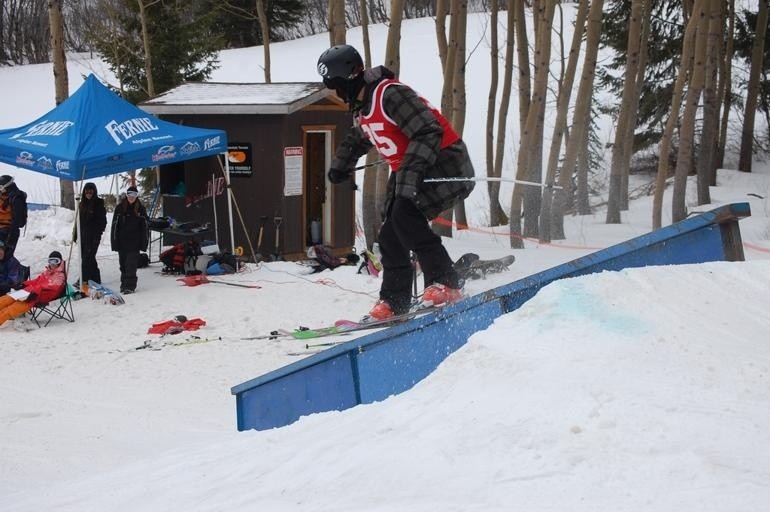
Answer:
[328,168,350,185]
[391,194,414,227]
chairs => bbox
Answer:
[29,260,75,328]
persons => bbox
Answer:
[0,250,65,327]
[109,186,150,294]
[0,241,27,301]
[0,174,26,256]
[71,180,108,290]
[313,43,478,325]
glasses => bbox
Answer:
[86,189,94,194]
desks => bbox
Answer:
[148,218,210,266]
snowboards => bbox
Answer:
[88,280,126,305]
[292,293,468,339]
[455,253,515,280]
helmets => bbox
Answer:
[317,44,364,81]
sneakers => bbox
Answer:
[368,297,410,320]
[421,281,466,307]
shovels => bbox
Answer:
[270,217,282,261]
[250,215,268,262]
